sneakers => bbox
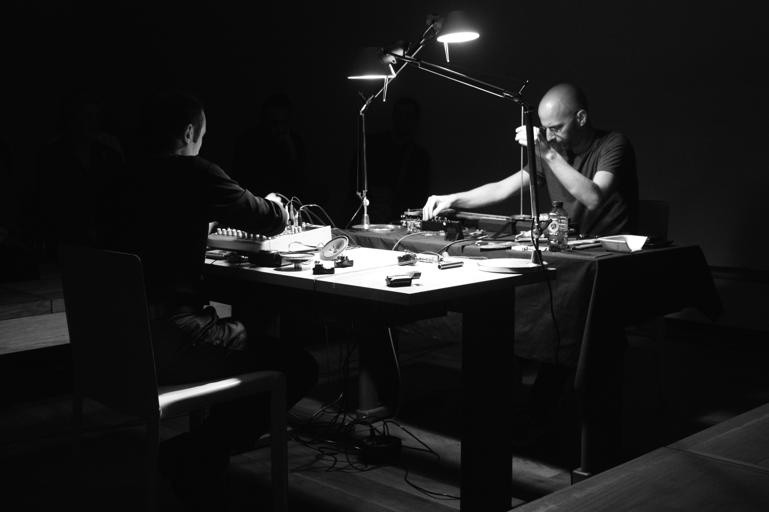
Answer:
[159,428,235,510]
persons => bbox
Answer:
[346,97,431,239]
[232,92,306,215]
[92,97,318,491]
[422,84,639,447]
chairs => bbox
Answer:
[633,198,675,425]
[56,237,291,510]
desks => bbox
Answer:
[206,242,558,510]
[0,279,72,355]
[665,402,765,471]
[339,224,702,484]
[510,445,767,511]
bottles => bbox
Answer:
[547,200,570,253]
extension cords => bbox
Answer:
[360,435,402,465]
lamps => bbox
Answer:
[350,9,481,231]
[345,40,550,274]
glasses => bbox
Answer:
[538,117,576,135]
